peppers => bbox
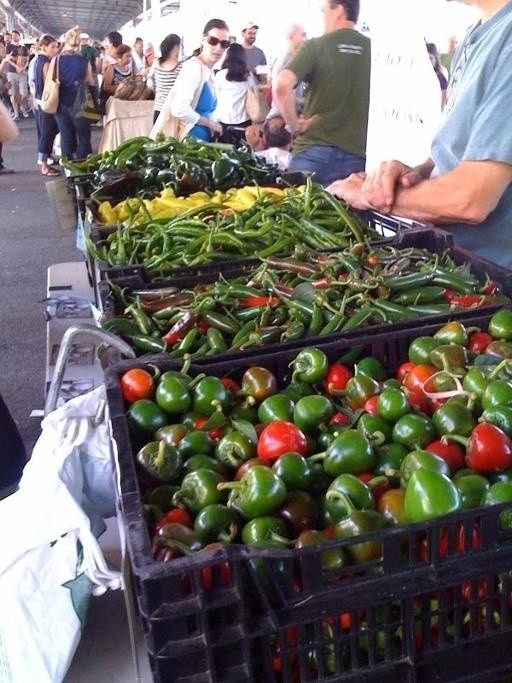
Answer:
[122,308,512,682]
[58,133,510,357]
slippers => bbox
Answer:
[41,167,60,176]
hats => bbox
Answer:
[241,21,259,32]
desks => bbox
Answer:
[99,96,154,153]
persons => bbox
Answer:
[326,0,511,270]
[273,0,371,184]
[2,18,458,176]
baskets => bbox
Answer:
[67,154,512,682]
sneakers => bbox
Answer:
[0,167,14,174]
[19,105,29,118]
[12,116,21,121]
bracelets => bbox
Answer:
[284,121,303,135]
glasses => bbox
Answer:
[206,36,230,48]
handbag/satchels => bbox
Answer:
[148,59,203,144]
[40,54,63,115]
[72,81,101,124]
